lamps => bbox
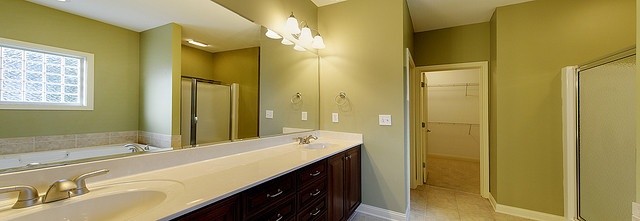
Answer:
[264,28,304,53]
[286,12,326,50]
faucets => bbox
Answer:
[124,142,150,154]
[304,134,318,144]
[0,188,168,221]
[42,178,78,205]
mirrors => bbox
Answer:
[0,0,327,175]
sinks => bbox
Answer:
[298,142,340,151]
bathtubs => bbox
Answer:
[0,142,164,170]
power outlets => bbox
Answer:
[267,111,273,118]
[331,113,339,123]
[380,114,391,125]
[303,112,306,119]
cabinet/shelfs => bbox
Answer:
[295,157,328,221]
[248,172,296,221]
[327,140,363,221]
[168,190,243,220]
